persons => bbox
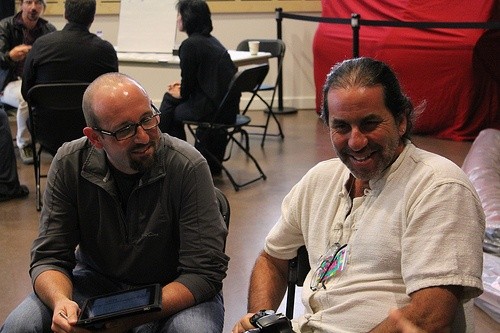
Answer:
[0,72,229,333]
[230,57,486,333]
[158,0,242,186]
[0,0,120,201]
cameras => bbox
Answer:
[250,308,296,333]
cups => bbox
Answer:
[248,41,260,55]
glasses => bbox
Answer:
[310,242,347,292]
[94,105,161,141]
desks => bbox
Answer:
[172,49,272,152]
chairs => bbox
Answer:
[236,39,286,148]
[181,64,270,191]
[26,83,91,212]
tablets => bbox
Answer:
[74,282,161,325]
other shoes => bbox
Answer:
[0,185,28,200]
[19,146,34,163]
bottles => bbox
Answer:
[96,30,102,38]
